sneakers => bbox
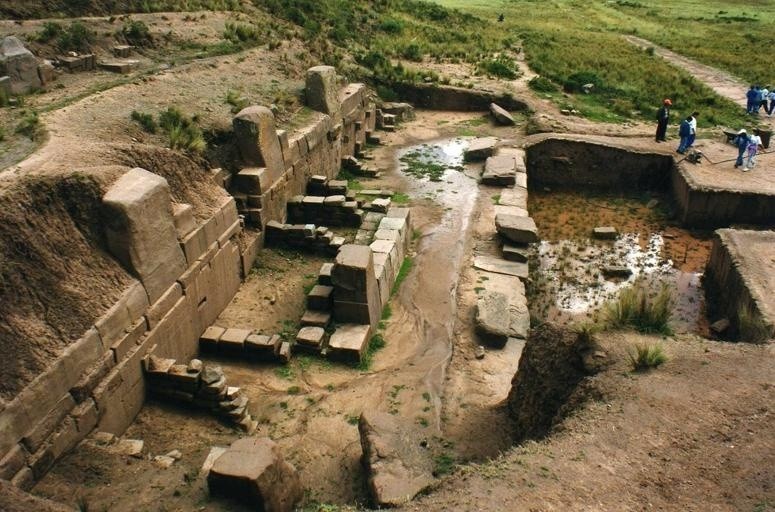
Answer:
[735,162,756,172]
[677,145,694,154]
[656,139,666,143]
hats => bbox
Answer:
[738,129,747,134]
[665,99,672,105]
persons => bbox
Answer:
[731,129,751,168]
[746,84,775,118]
[742,128,768,170]
[685,112,700,151]
[656,99,672,143]
[677,116,693,154]
